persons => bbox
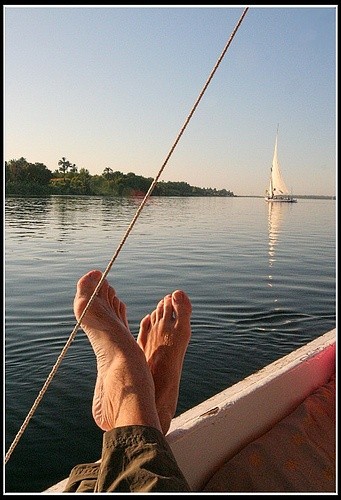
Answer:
[65,267,196,493]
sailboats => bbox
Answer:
[263,129,294,203]
